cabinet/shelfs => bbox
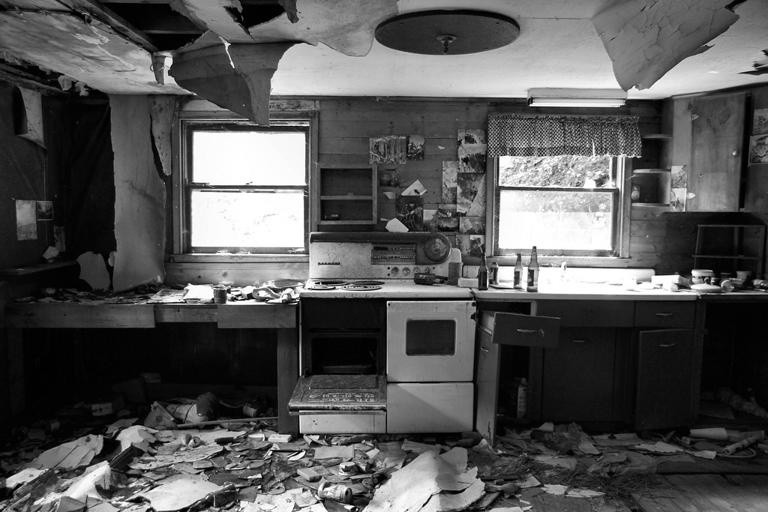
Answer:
[693,224,762,284]
[477,299,560,451]
[631,88,767,216]
[538,299,635,425]
[316,161,379,226]
[617,301,703,435]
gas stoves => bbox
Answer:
[300,278,385,297]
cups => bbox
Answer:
[214,286,226,304]
[447,262,463,284]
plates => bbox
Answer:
[691,284,720,292]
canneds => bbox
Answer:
[318,482,353,503]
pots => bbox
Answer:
[414,273,448,285]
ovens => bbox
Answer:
[288,297,386,415]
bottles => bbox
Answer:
[280,288,293,304]
[514,253,523,288]
[489,262,498,284]
[527,245,539,292]
[478,254,487,290]
